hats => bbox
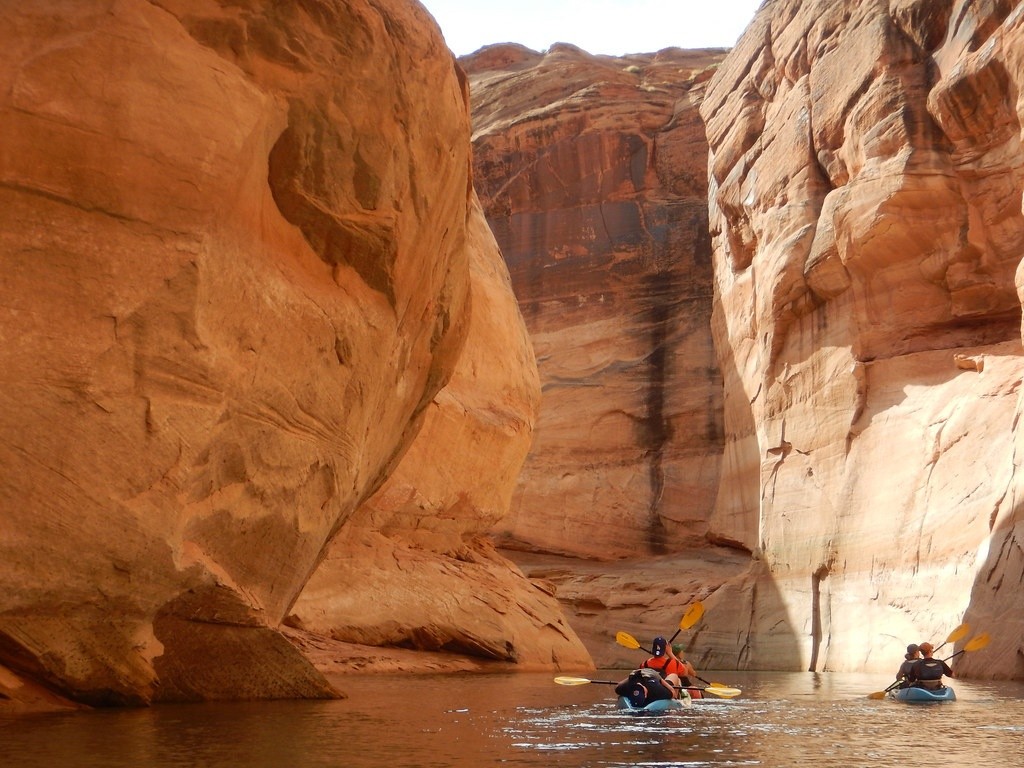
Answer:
[652,637,667,655]
[672,641,687,655]
[630,684,645,706]
[920,642,934,655]
[907,644,921,652]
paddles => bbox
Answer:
[552,675,744,700]
[868,623,972,699]
[669,600,707,643]
[867,633,993,702]
[615,631,731,688]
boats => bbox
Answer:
[889,684,956,702]
[616,687,704,712]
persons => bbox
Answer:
[908,641,956,691]
[637,636,685,691]
[659,642,697,691]
[896,643,928,688]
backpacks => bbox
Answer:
[644,658,672,679]
